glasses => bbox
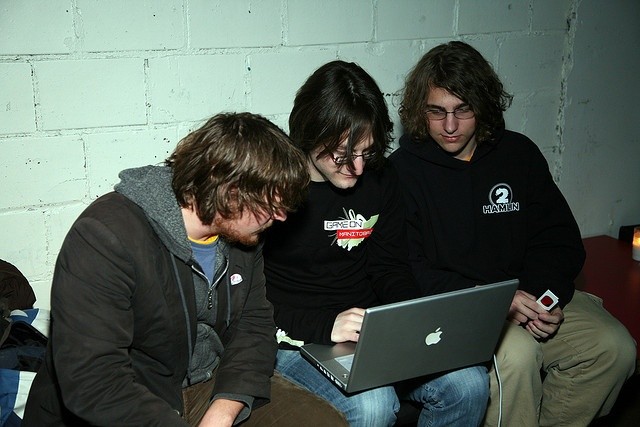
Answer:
[425,108,475,120]
[322,143,378,166]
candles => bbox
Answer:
[633,229,640,262]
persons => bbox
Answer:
[387,40,637,427]
[261,63,490,427]
[23,112,350,427]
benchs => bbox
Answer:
[195,233,640,427]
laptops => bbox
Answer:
[300,279,519,393]
